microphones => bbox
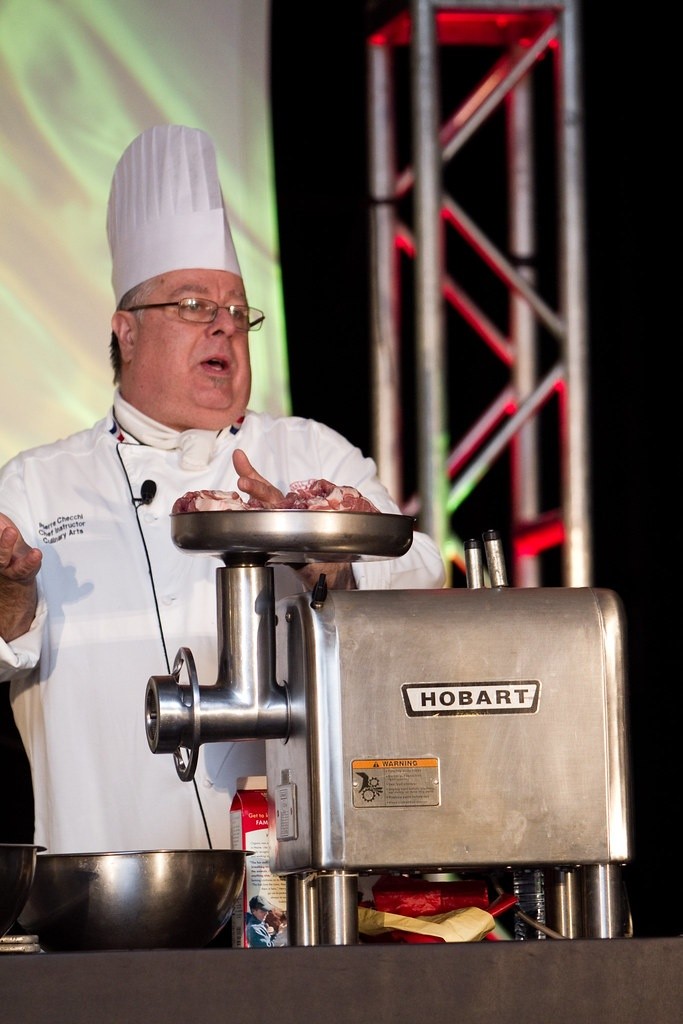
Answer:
[130,478,157,510]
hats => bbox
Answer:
[104,127,242,305]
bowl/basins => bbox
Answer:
[0,843,47,941]
[32,848,254,951]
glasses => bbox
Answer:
[128,296,265,332]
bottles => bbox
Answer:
[514,868,546,941]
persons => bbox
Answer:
[0,124,447,857]
[244,896,287,947]
